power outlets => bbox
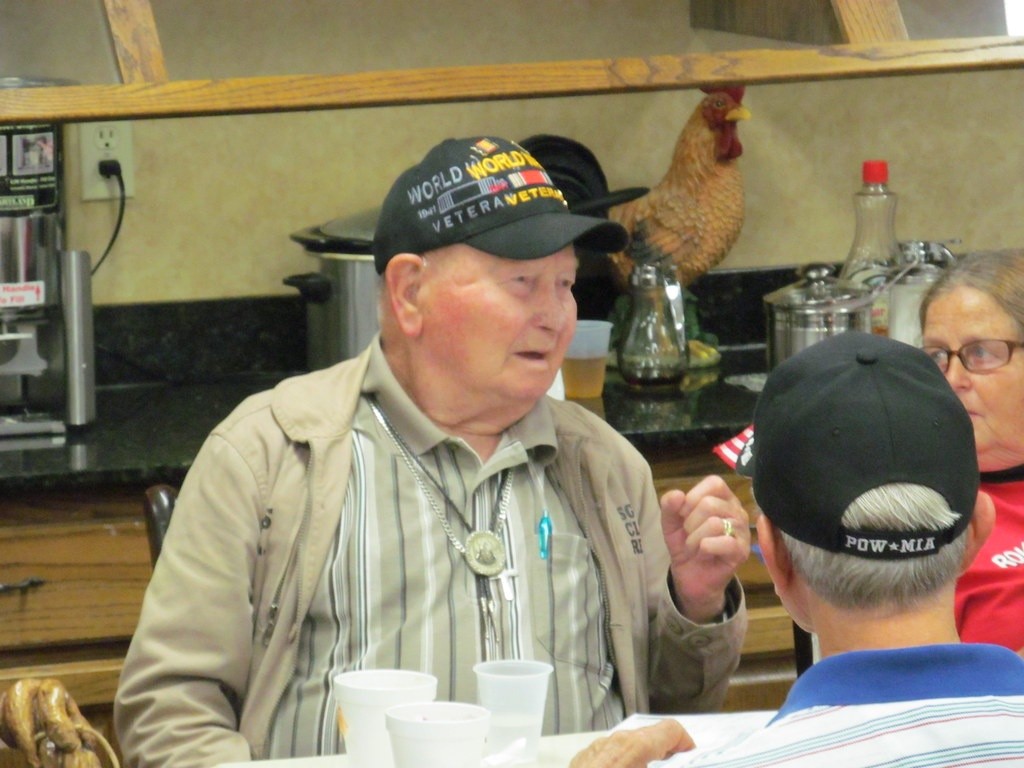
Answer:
[78,120,135,201]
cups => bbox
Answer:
[384,702,489,768]
[562,320,612,398]
[760,265,873,378]
[334,669,437,755]
[473,660,553,762]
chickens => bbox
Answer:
[606,83,752,369]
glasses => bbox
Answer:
[924,336,1024,375]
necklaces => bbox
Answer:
[365,395,517,638]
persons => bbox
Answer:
[113,136,750,768]
[570,330,1024,768]
[917,248,1024,657]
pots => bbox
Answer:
[281,210,387,374]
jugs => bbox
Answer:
[619,262,689,390]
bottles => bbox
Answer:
[838,160,901,286]
[888,240,957,351]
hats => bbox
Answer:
[737,330,981,562]
[375,134,630,274]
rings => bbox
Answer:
[722,519,734,536]
